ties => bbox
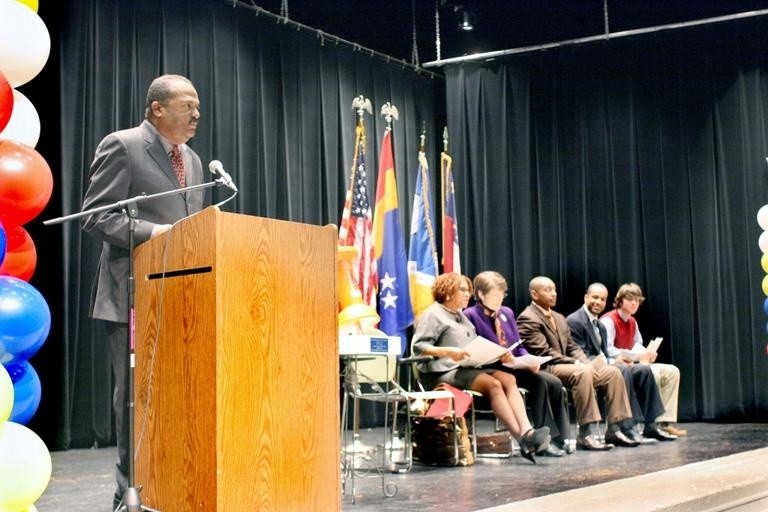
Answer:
[171,144,185,187]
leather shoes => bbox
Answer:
[660,425,687,436]
[624,429,656,445]
[535,444,565,457]
[605,430,640,447]
[643,428,678,441]
[576,435,615,451]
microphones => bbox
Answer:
[208,160,239,193]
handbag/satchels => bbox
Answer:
[477,431,512,454]
[410,387,474,466]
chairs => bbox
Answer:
[340,328,644,505]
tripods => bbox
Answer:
[42,183,214,512]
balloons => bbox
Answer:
[756,205,768,354]
[0,0,54,512]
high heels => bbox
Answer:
[519,426,552,464]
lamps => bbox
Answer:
[448,1,478,32]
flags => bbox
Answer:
[337,127,379,331]
[372,127,415,359]
[441,151,462,274]
[406,152,439,328]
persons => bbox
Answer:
[566,282,677,444]
[412,272,553,464]
[462,270,573,456]
[79,74,204,504]
[516,276,641,450]
[598,282,687,437]
